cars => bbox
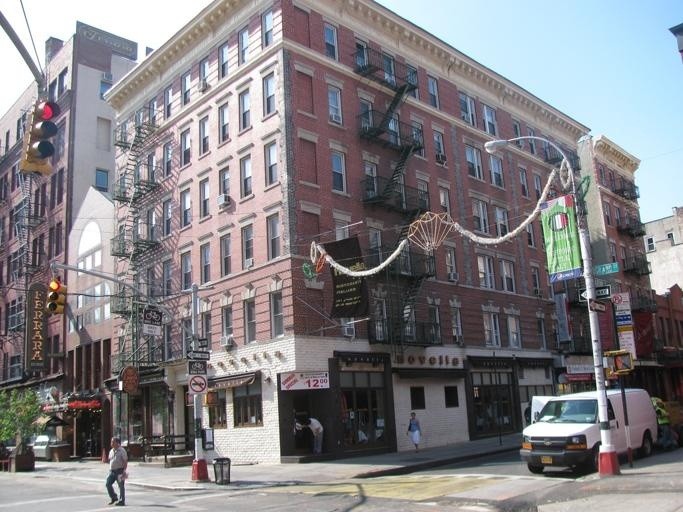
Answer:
[34,434,67,445]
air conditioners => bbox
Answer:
[340,325,356,338]
[219,336,233,346]
[196,80,207,93]
[454,335,464,343]
[448,272,459,281]
[215,194,231,206]
[434,152,447,165]
[243,257,254,269]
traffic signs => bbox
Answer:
[591,262,619,276]
[186,350,209,360]
[189,338,207,350]
[587,299,607,313]
[578,284,610,302]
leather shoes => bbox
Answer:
[109,499,125,506]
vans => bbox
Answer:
[519,388,658,475]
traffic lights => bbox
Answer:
[53,282,67,316]
[610,350,633,373]
[44,278,60,313]
[27,98,61,165]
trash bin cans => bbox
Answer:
[212,457,231,485]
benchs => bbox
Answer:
[0,460,9,472]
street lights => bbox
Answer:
[483,136,620,477]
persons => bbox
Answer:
[406,412,423,453]
[105,437,128,506]
[656,400,670,447]
[302,417,324,455]
